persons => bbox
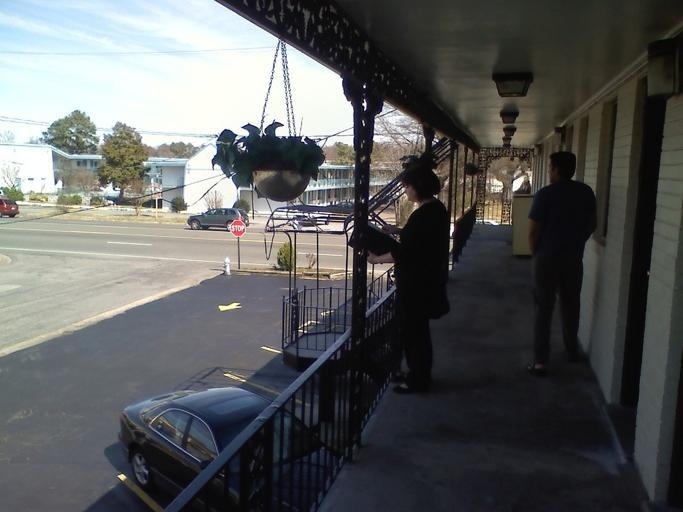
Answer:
[526,149,598,375]
[379,157,451,393]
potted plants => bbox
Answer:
[208,121,327,204]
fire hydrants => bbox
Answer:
[223,257,232,275]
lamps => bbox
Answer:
[492,70,533,151]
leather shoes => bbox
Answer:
[391,371,430,393]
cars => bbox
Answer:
[187,208,250,231]
[287,202,353,226]
[118,386,340,512]
[0,198,20,218]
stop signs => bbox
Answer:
[231,220,246,237]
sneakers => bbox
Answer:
[526,358,549,377]
[564,347,584,361]
